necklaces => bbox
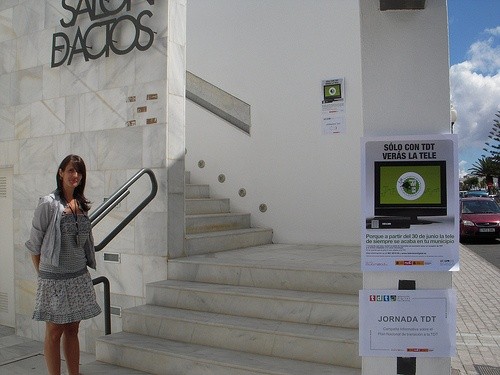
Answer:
[64,196,85,247]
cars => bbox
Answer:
[458,197,500,242]
[459,189,500,199]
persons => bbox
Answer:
[26,153,102,374]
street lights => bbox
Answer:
[449,104,457,134]
